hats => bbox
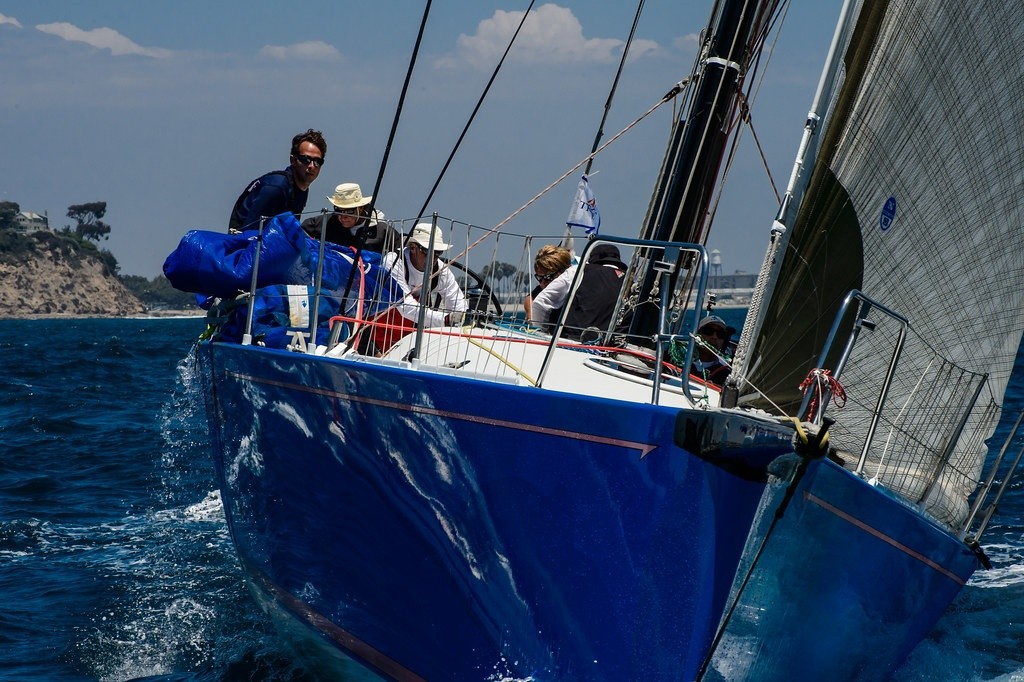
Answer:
[403,223,454,252]
[325,183,373,209]
[588,244,628,271]
[693,315,728,338]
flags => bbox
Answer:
[566,173,601,238]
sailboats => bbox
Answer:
[189,0,1024,682]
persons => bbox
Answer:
[227,128,327,234]
[366,223,467,357]
[315,183,388,242]
[523,244,629,344]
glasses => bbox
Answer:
[697,325,728,340]
[413,242,443,257]
[333,205,357,214]
[296,155,324,168]
[534,268,560,282]
